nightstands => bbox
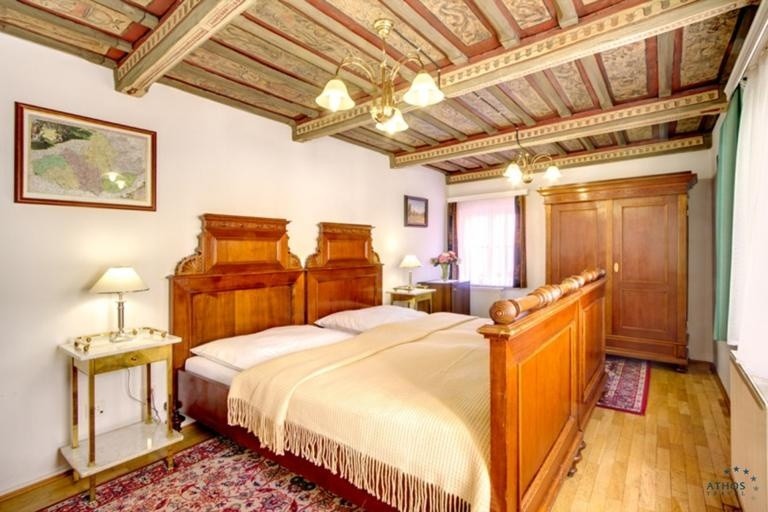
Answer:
[58,328,184,501]
[386,287,435,314]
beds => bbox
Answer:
[167,213,610,512]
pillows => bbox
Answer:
[313,304,429,333]
[190,324,354,372]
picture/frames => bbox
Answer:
[404,195,428,227]
[15,102,157,212]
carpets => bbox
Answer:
[597,352,651,415]
[36,435,369,512]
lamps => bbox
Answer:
[90,266,150,343]
[504,150,562,186]
[316,19,444,133]
[394,254,421,290]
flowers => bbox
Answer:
[431,251,458,267]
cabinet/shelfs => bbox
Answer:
[538,170,697,372]
[418,280,470,314]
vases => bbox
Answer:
[440,264,448,281]
